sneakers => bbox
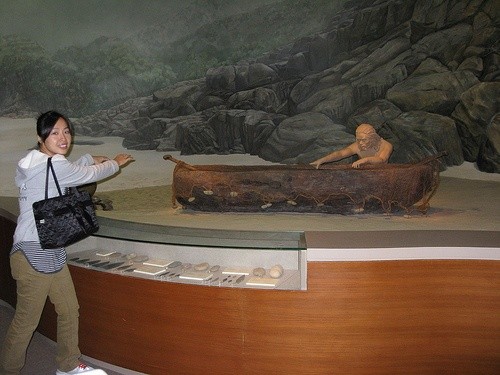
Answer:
[56,364,108,375]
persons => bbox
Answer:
[1,111,134,375]
[309,122,394,169]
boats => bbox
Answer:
[163,152,446,217]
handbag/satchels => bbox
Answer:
[33,157,99,248]
